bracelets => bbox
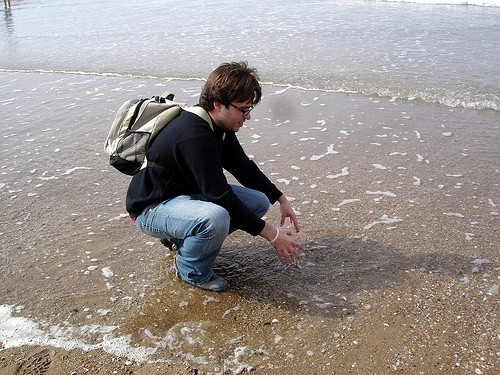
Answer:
[270,229,280,243]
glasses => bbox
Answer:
[229,102,253,116]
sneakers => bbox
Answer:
[176,270,228,292]
[159,237,180,251]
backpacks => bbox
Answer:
[104,91,214,176]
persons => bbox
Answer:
[125,62,305,293]
